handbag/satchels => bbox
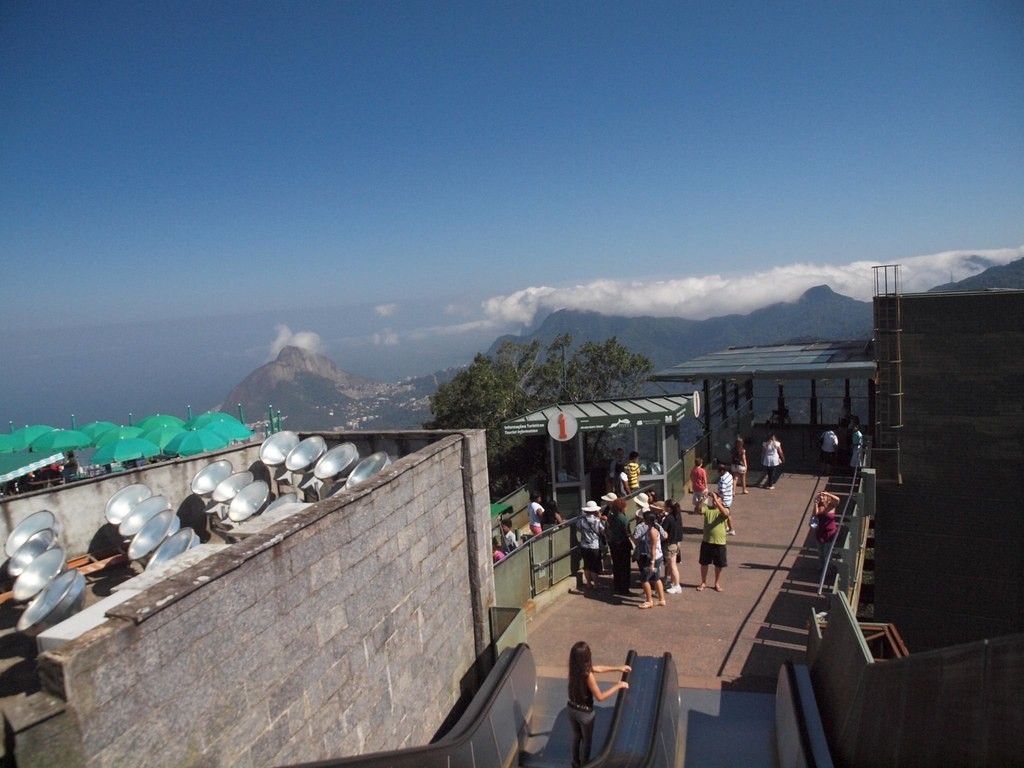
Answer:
[809,515,819,530]
[819,432,827,445]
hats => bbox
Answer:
[601,492,617,501]
[635,507,651,519]
[633,493,649,508]
[650,501,665,509]
[581,501,601,511]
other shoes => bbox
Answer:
[638,601,654,608]
[652,592,659,597]
[744,490,749,494]
[666,585,681,594]
[769,486,775,489]
[727,530,735,535]
[639,592,646,597]
[654,600,666,606]
[665,584,673,590]
[593,585,602,589]
[726,527,729,531]
[587,584,592,589]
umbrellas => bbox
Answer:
[0,410,254,484]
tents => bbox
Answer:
[0,452,65,497]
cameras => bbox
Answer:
[708,491,715,497]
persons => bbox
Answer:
[694,491,730,592]
[0,451,78,499]
[527,491,564,539]
[690,458,709,515]
[820,425,839,475]
[638,511,666,609]
[576,489,683,598]
[849,425,864,476]
[609,448,640,498]
[761,432,785,490]
[814,491,840,575]
[716,464,735,535]
[567,641,631,768]
[730,438,749,498]
[492,519,518,564]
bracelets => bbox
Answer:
[651,561,655,563]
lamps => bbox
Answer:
[5,429,392,637]
